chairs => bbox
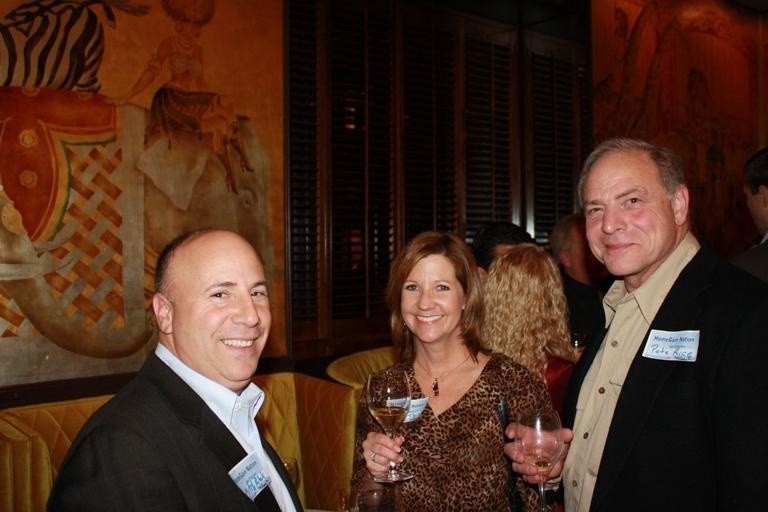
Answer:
[327,345,398,389]
[0,372,360,512]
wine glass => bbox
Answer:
[363,368,413,484]
[514,406,564,511]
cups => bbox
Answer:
[349,476,405,511]
[280,456,300,490]
[571,335,591,361]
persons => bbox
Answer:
[356,139,767,512]
[46,230,305,512]
